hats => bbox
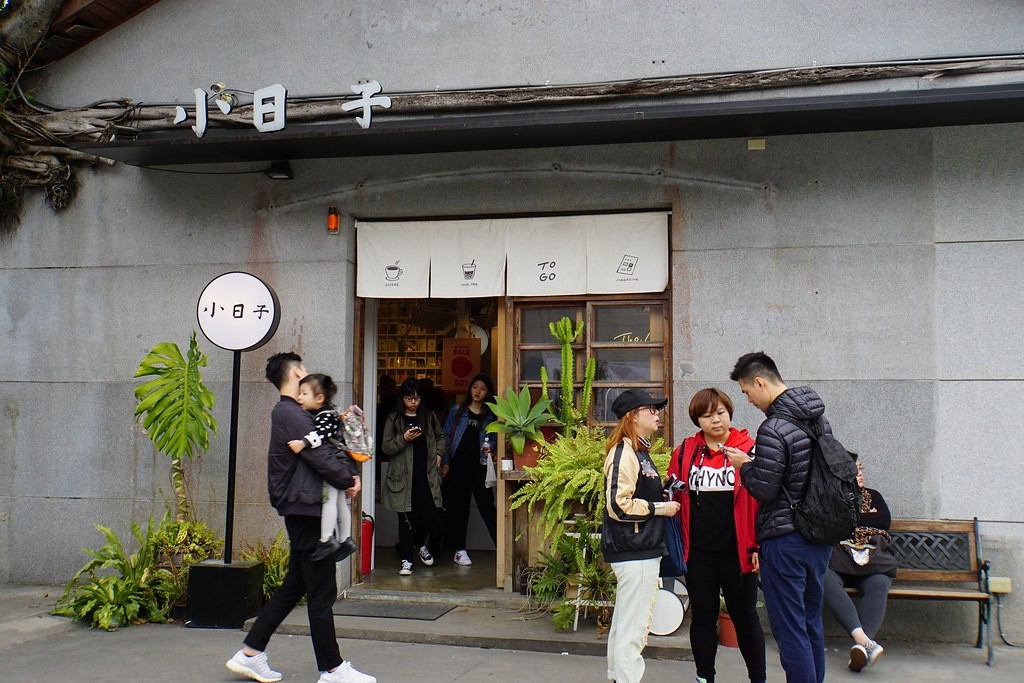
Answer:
[611,389,668,420]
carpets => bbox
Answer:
[332,598,458,620]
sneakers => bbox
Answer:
[226,649,282,682]
[847,645,868,672]
[310,536,341,562]
[318,660,377,683]
[329,538,357,562]
[399,560,413,575]
[865,641,884,664]
[454,549,472,565]
[417,546,434,565]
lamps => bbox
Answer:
[265,159,293,178]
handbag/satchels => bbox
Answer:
[485,452,497,489]
[659,514,687,577]
[831,536,898,579]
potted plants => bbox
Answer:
[45,328,223,633]
[719,594,739,647]
[485,316,673,629]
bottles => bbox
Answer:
[479,436,491,466]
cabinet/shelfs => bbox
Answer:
[377,298,440,386]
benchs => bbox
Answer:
[845,516,996,668]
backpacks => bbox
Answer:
[768,413,864,547]
[314,404,371,463]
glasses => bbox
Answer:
[404,396,420,401]
[637,405,655,415]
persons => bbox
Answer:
[601,389,681,683]
[225,352,377,683]
[823,450,891,672]
[723,353,835,683]
[377,375,497,574]
[666,389,767,683]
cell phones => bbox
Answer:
[410,428,420,434]
[663,473,677,492]
